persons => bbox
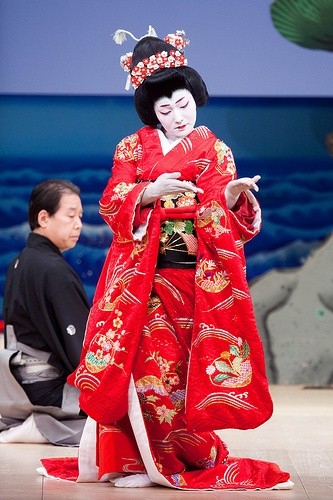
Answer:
[0,179,93,448]
[36,34,297,492]
